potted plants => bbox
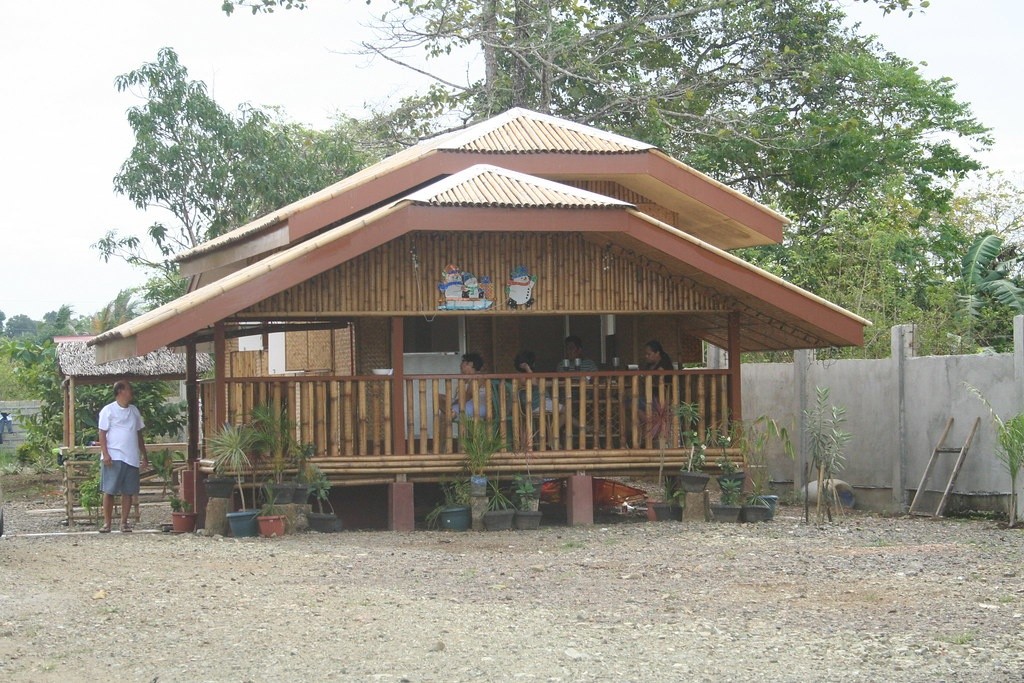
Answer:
[250,478,289,537]
[513,472,542,530]
[295,441,315,504]
[511,428,541,510]
[455,416,510,496]
[710,416,794,523]
[653,476,683,522]
[202,455,236,499]
[424,474,472,532]
[483,470,514,531]
[305,463,338,534]
[246,398,300,505]
[199,418,267,538]
[170,498,197,532]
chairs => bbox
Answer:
[460,380,515,445]
[518,385,552,450]
[635,381,682,450]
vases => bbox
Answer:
[678,469,710,492]
[716,472,745,494]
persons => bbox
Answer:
[438,353,486,451]
[98,379,148,532]
[514,351,591,449]
[623,341,673,448]
[556,336,596,399]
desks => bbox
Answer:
[548,380,632,448]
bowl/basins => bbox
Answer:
[284,371,304,376]
[371,369,393,375]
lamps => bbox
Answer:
[411,242,419,268]
[602,248,611,271]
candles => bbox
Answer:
[574,357,581,367]
[563,358,570,368]
[611,357,620,367]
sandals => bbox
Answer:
[120,521,133,532]
[98,522,111,533]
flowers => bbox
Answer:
[706,425,737,475]
[675,402,707,472]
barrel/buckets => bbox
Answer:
[801,478,856,508]
[769,479,794,501]
